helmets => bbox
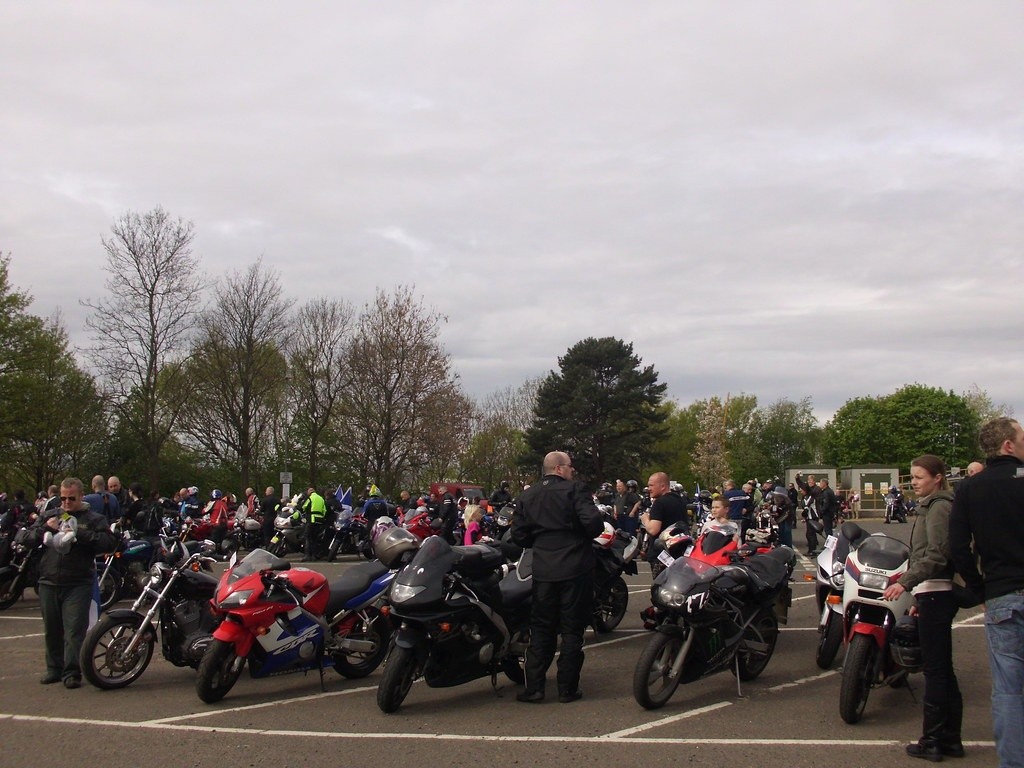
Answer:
[601,482,613,493]
[888,485,896,493]
[659,521,694,558]
[669,483,683,493]
[889,615,923,674]
[210,489,222,501]
[188,486,199,496]
[376,527,422,570]
[626,480,637,493]
[698,489,713,505]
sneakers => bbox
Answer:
[65,675,81,688]
[40,674,62,684]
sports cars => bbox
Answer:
[838,521,922,726]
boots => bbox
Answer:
[937,692,964,757]
[906,688,942,761]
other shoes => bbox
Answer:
[300,558,312,562]
[559,688,583,703]
[902,520,907,523]
[516,688,544,701]
[802,550,817,556]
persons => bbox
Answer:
[670,478,792,548]
[836,487,859,520]
[948,416,1024,768]
[881,484,908,524]
[953,462,984,496]
[0,475,343,688]
[510,451,605,703]
[361,480,514,540]
[787,471,836,556]
[883,455,965,762]
[614,471,689,629]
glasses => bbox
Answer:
[60,492,82,502]
[553,462,574,470]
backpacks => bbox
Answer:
[927,496,986,608]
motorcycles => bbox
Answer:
[634,524,799,710]
[803,521,889,668]
[881,493,907,524]
[1,504,646,714]
